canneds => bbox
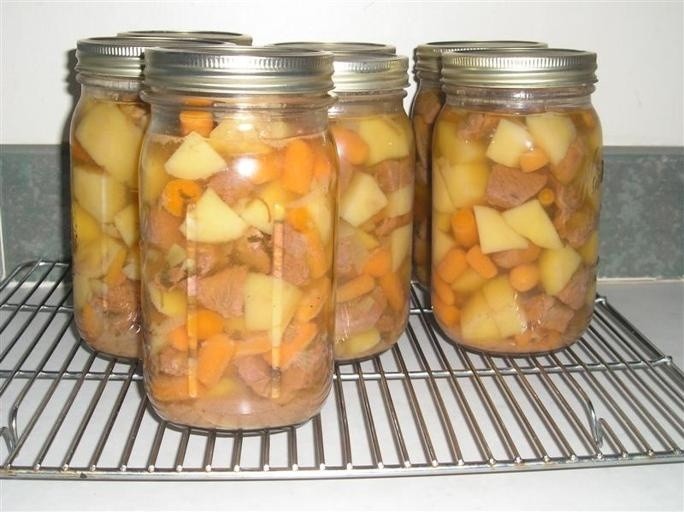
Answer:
[429,47,605,358]
[409,40,550,289]
[139,46,338,433]
[334,52,416,366]
[69,37,242,363]
